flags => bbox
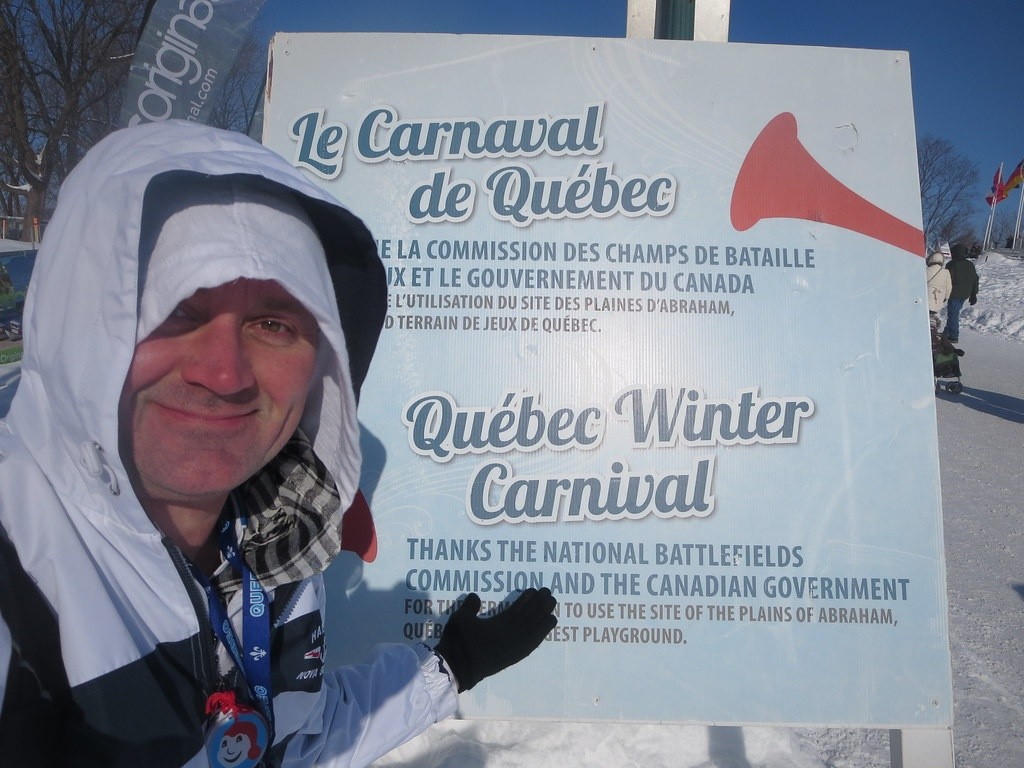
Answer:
[985,164,1008,206]
[1005,159,1024,193]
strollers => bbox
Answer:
[930,316,966,396]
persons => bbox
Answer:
[0,124,557,768]
[927,245,979,344]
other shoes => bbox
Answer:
[947,336,958,342]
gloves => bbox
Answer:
[968,295,978,306]
[433,587,558,694]
